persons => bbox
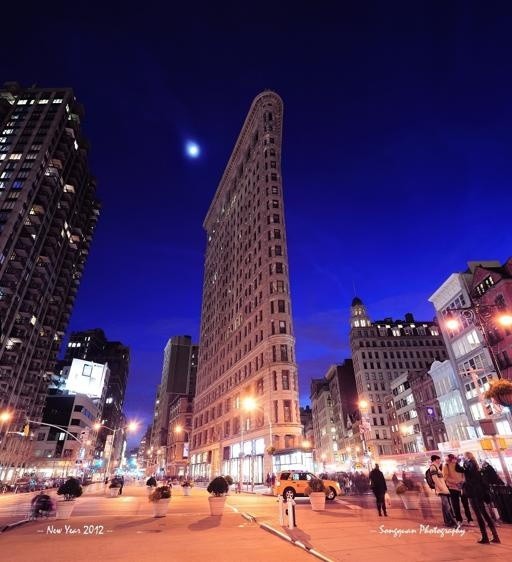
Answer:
[430,451,501,544]
[321,471,368,491]
[267,472,275,488]
[392,471,406,486]
[369,463,389,516]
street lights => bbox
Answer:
[442,302,512,414]
[175,426,190,443]
[242,397,273,446]
[94,422,137,481]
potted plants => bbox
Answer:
[147,474,235,517]
[108,479,122,497]
[395,477,423,511]
[306,477,327,510]
[54,475,83,520]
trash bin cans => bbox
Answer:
[492,486,512,523]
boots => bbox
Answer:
[478,525,490,545]
[489,521,501,543]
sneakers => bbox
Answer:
[456,519,475,528]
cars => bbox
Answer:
[274,470,341,501]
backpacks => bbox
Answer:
[425,465,439,489]
